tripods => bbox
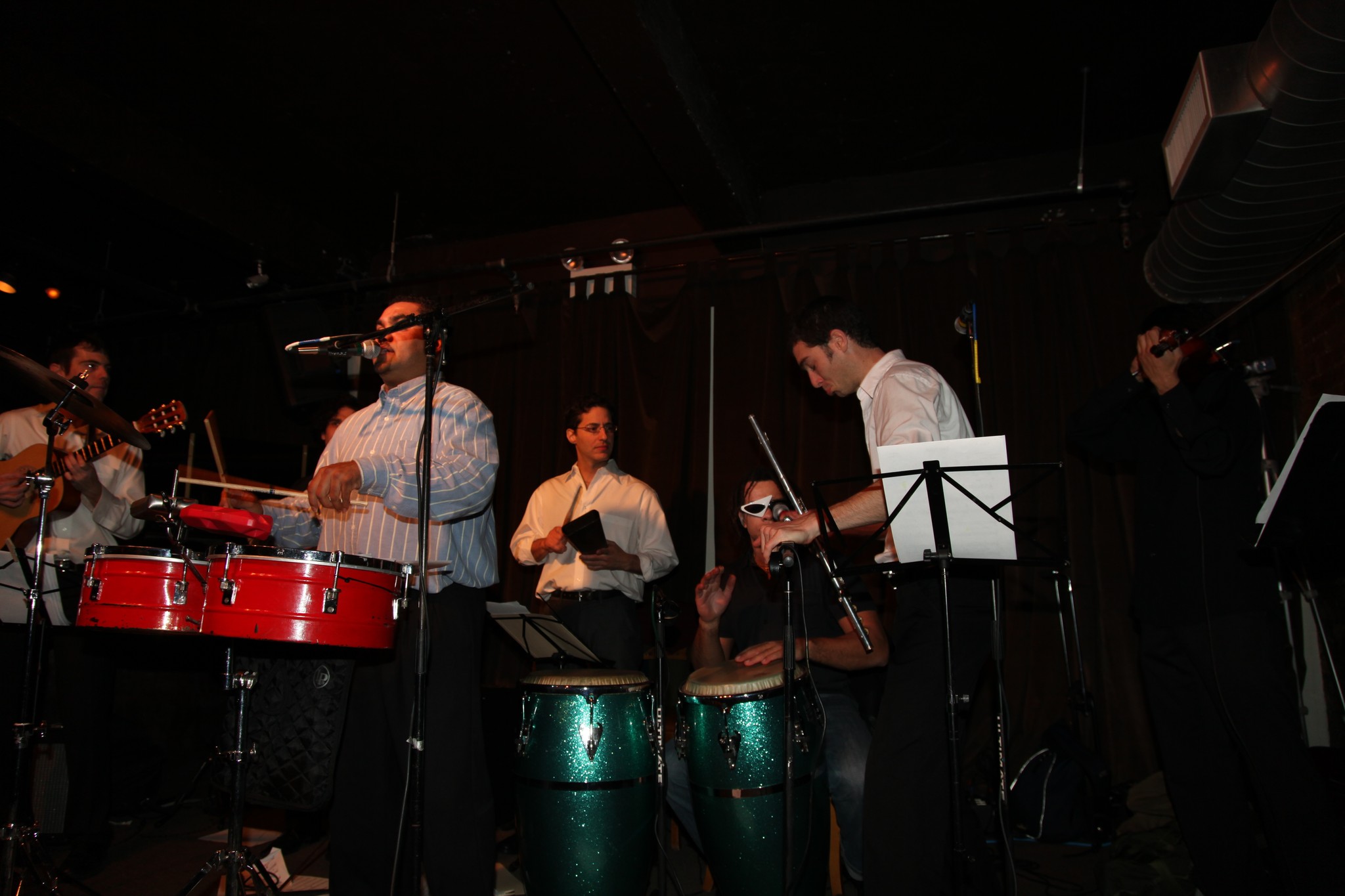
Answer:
[173,639,282,896]
[0,393,70,896]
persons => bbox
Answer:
[507,397,679,675]
[291,393,359,493]
[657,463,889,896]
[751,315,998,896]
[1,333,148,895]
[1063,308,1340,896]
[213,299,506,896]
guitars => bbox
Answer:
[0,399,188,554]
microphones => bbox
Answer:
[955,300,975,335]
[773,505,789,521]
[291,340,380,359]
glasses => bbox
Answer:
[573,424,618,434]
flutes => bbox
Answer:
[747,413,875,654]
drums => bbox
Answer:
[201,536,412,649]
[679,662,819,896]
[513,670,664,896]
[72,541,209,631]
